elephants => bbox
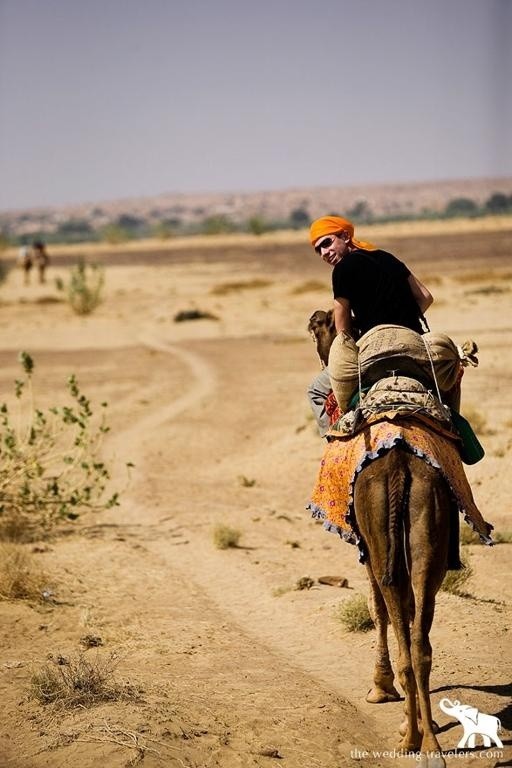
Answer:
[439,698,504,749]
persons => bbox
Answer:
[33,242,47,283]
[307,216,461,438]
[18,241,33,283]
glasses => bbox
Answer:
[314,238,336,253]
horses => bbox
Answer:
[307,306,468,768]
